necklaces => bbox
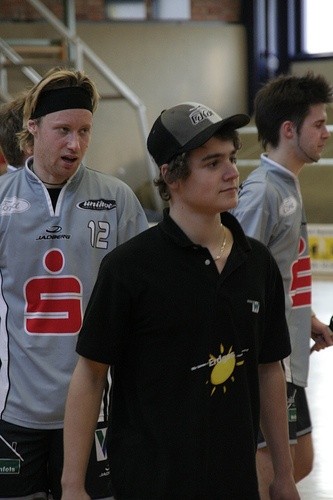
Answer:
[213,223,227,261]
[42,179,68,186]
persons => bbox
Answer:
[229,70,333,500]
[63,103,302,500]
[0,95,34,173]
[0,66,149,500]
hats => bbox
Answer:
[148,103,250,170]
